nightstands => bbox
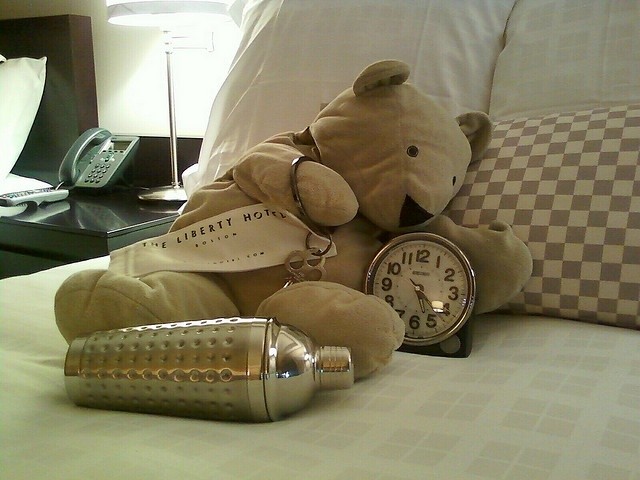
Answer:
[0,183,182,277]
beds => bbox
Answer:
[1,253,639,480]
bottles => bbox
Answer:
[65,315,354,422]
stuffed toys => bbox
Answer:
[54,60,533,382]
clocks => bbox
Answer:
[362,231,474,359]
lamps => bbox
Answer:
[107,1,234,204]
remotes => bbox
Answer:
[0,188,69,207]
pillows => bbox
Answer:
[443,107,639,332]
[0,54,49,190]
[484,0,640,113]
[182,0,519,192]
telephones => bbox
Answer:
[58,128,140,195]
